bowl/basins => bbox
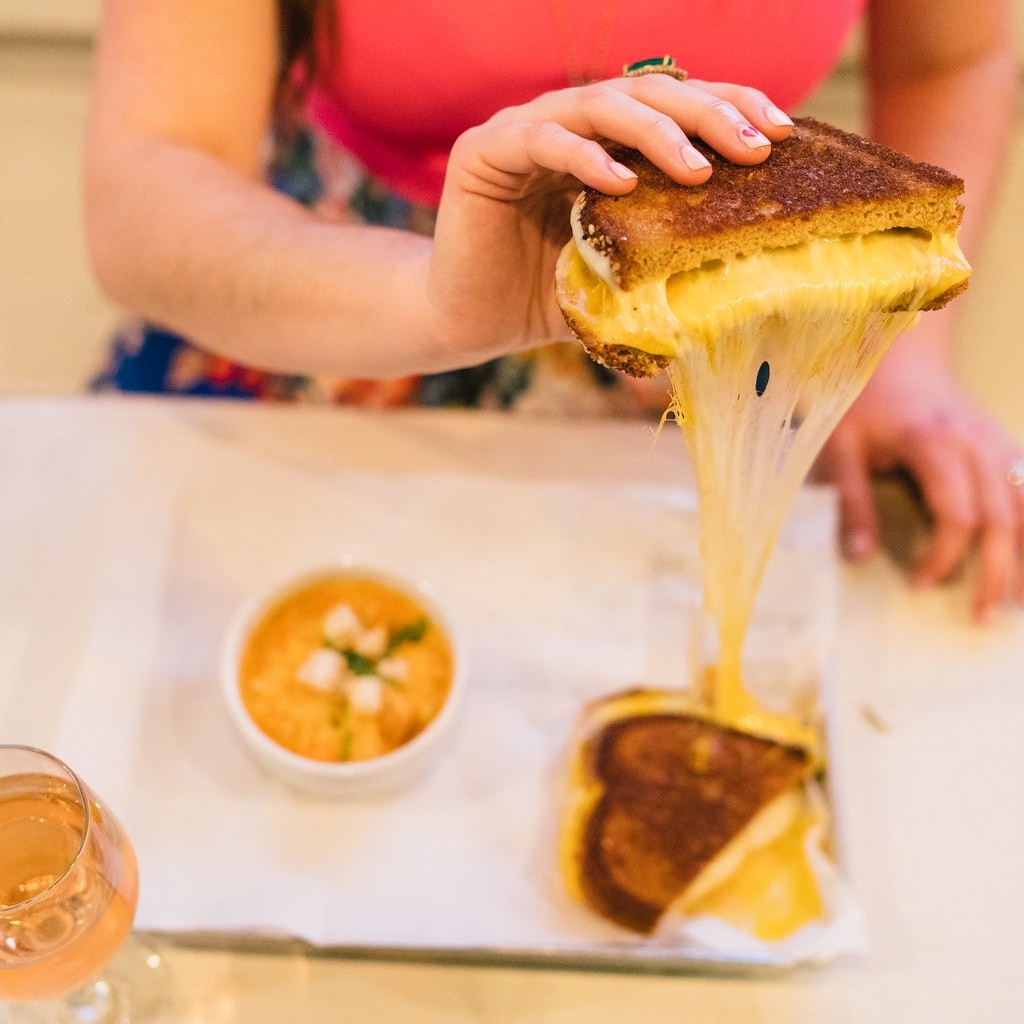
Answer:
[220,558,458,798]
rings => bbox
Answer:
[622,55,685,81]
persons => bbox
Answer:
[83,0,1023,626]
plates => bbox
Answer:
[58,419,851,974]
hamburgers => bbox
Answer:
[551,116,978,967]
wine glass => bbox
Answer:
[0,744,139,1024]
